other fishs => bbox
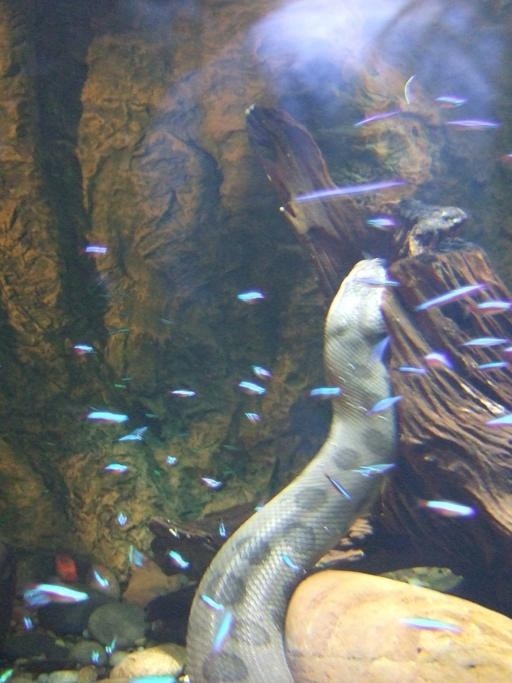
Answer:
[21,74,512,683]
